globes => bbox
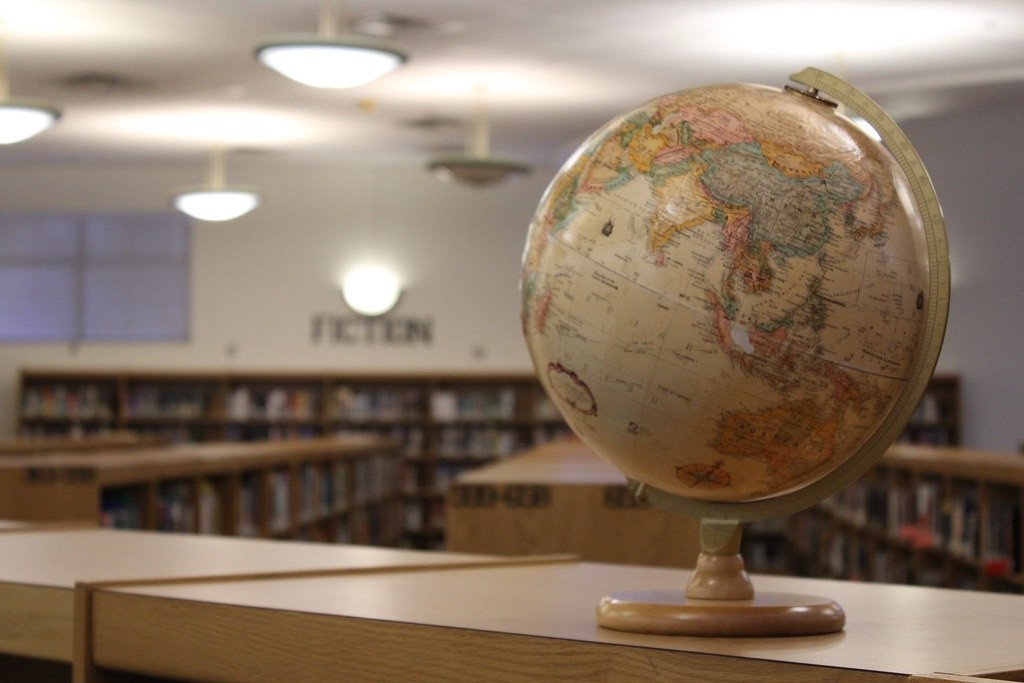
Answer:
[517,66,953,639]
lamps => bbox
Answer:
[0,71,62,146]
[429,108,536,190]
[173,150,261,233]
[252,8,411,92]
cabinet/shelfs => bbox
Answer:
[13,363,1024,593]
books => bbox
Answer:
[14,379,575,552]
[742,386,1023,599]
